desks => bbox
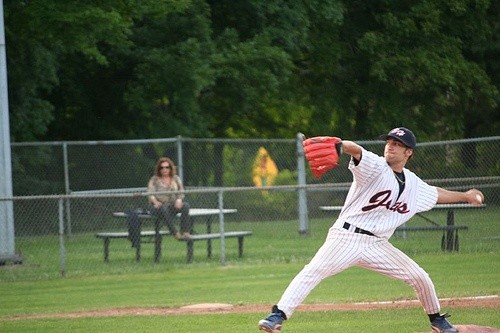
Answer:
[319,205,487,252]
[112,208,236,262]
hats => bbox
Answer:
[379,126,417,148]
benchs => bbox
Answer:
[180,230,253,262]
[390,223,469,252]
[93,229,172,264]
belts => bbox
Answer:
[342,222,374,235]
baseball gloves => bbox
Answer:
[303,135,343,177]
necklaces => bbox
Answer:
[391,167,408,185]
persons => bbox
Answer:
[148,156,193,240]
[259,127,484,333]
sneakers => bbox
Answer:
[258,305,285,333]
[431,311,459,333]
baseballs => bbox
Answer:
[471,194,483,206]
